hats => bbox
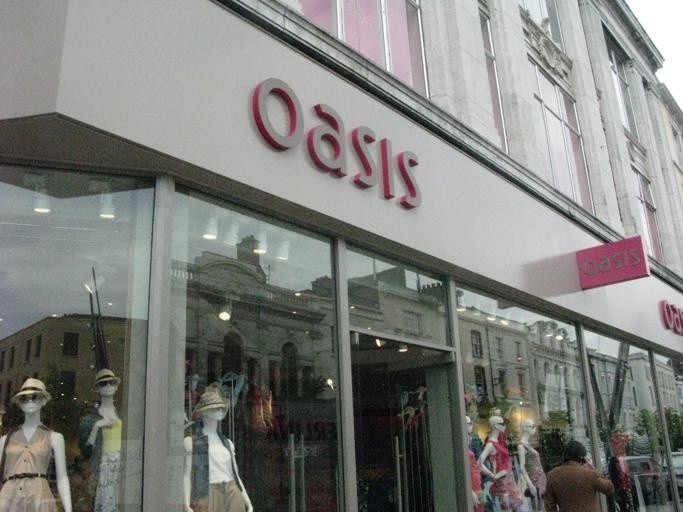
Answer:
[195,391,226,412]
[9,378,52,404]
[92,368,122,387]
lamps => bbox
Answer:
[202,203,290,260]
[376,338,408,352]
[457,293,564,341]
[218,299,233,320]
[84,273,110,295]
[99,184,116,218]
[34,183,51,213]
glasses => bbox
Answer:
[18,395,42,405]
[467,422,473,425]
[99,380,118,388]
[496,422,505,427]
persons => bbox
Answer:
[544,441,616,512]
[181,390,252,511]
[76,367,125,511]
[0,376,73,511]
[463,402,549,511]
[607,457,634,512]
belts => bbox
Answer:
[3,472,47,484]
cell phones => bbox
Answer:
[580,456,585,463]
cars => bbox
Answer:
[602,451,683,508]
